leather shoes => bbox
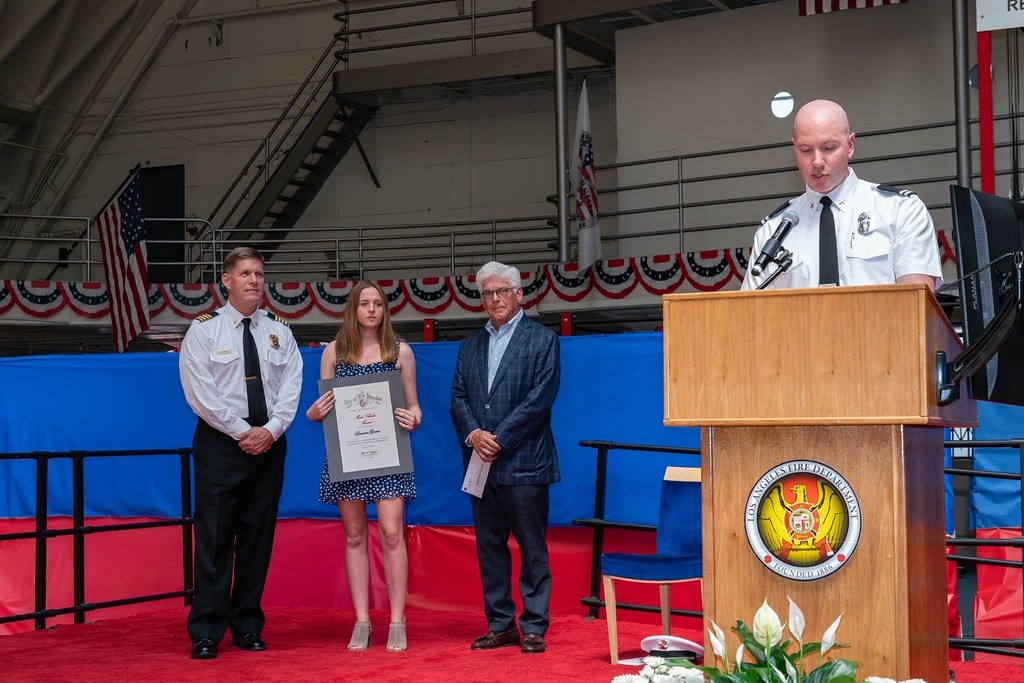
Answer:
[232,632,265,650]
[471,627,520,650]
[191,637,217,657]
[522,633,545,653]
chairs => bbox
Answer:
[601,467,701,665]
[324,252,363,281]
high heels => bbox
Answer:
[387,622,407,652]
[348,619,373,650]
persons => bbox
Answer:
[450,262,560,653]
[179,247,303,659]
[306,280,422,652]
[740,99,944,291]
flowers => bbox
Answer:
[610,592,927,683]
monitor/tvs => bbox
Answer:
[948,184,1024,406]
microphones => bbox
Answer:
[752,211,800,276]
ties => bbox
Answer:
[240,317,268,427]
[819,196,839,286]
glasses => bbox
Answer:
[481,287,515,300]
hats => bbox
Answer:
[640,635,705,665]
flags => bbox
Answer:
[97,167,149,354]
[570,78,602,276]
